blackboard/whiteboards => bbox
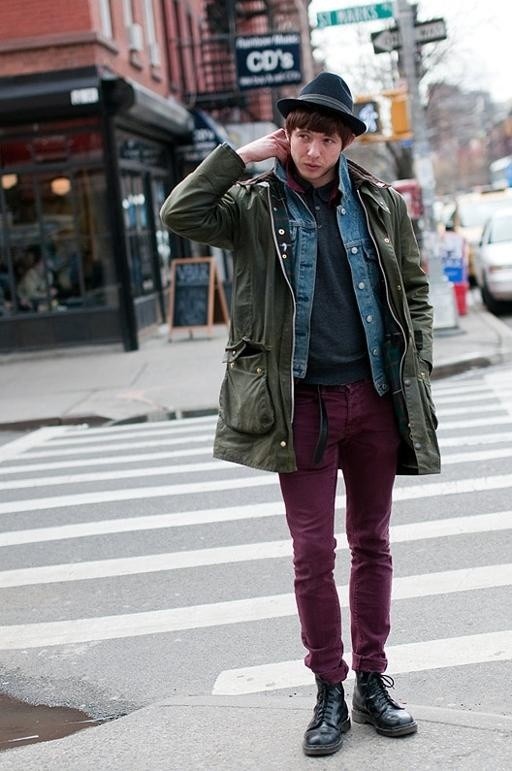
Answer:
[168,256,216,332]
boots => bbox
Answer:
[301,675,351,758]
[351,668,418,738]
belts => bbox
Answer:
[294,380,340,467]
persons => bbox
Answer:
[16,254,67,313]
[438,221,470,315]
[160,87,442,759]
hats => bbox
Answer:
[275,71,367,138]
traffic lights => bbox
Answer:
[355,99,382,139]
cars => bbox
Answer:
[435,186,512,315]
